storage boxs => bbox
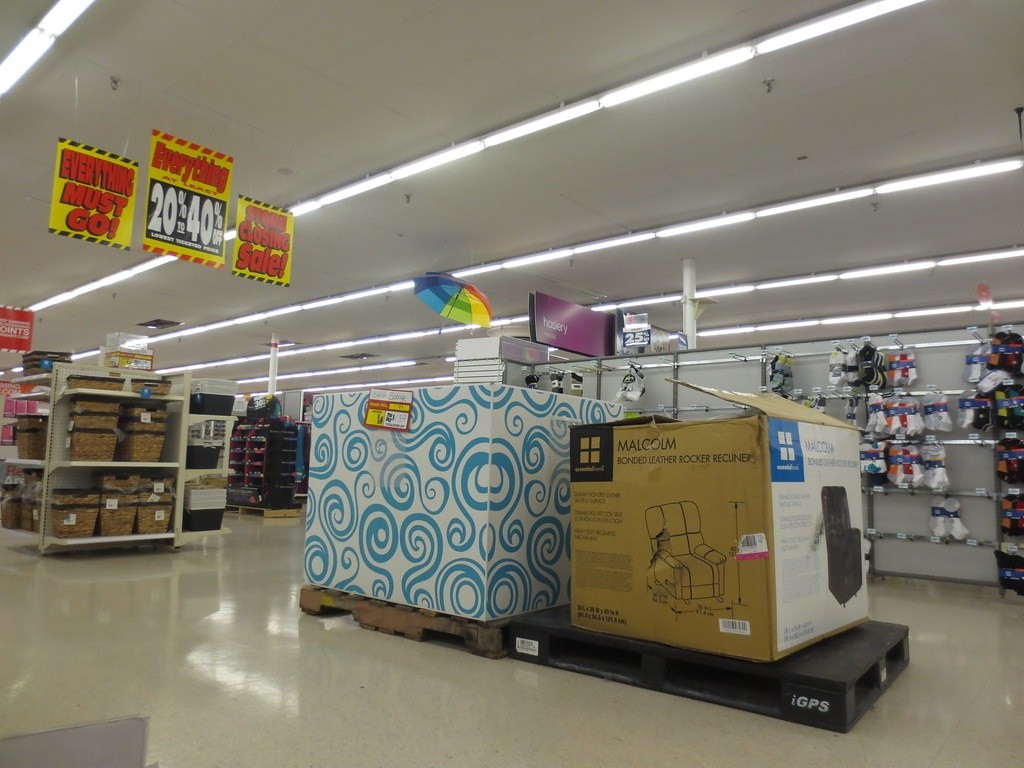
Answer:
[569,378,868,663]
[304,383,623,622]
[457,335,549,366]
[182,378,237,531]
[97,347,153,370]
[106,332,149,350]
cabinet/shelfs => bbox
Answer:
[226,415,311,508]
[0,362,190,553]
[532,320,1024,596]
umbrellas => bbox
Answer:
[412,271,492,334]
